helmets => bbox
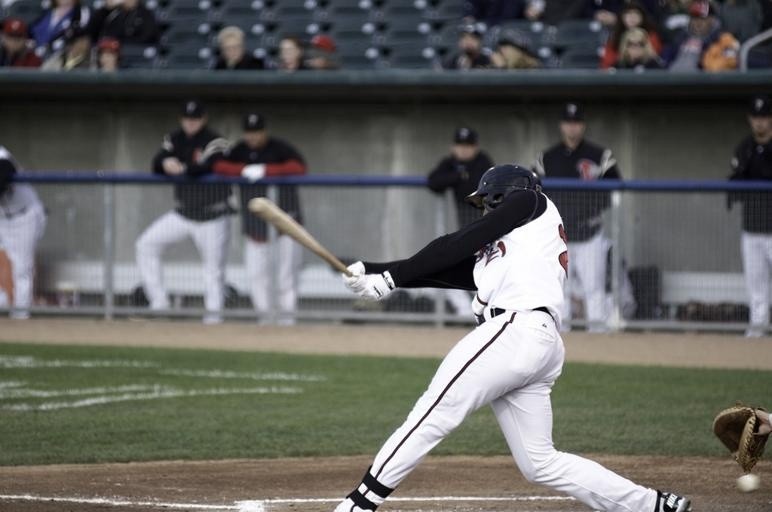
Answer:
[464,164,541,208]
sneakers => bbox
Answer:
[659,492,691,512]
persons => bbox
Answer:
[210,25,311,72]
[752,409,772,436]
[427,126,495,314]
[215,114,308,330]
[435,0,744,69]
[0,146,47,320]
[1,1,165,73]
[726,99,772,340]
[435,0,772,70]
[534,104,626,334]
[135,99,238,326]
[334,164,691,512]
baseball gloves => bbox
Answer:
[712,404,767,473]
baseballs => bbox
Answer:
[737,473,758,493]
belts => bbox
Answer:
[478,308,506,323]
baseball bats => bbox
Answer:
[248,198,355,279]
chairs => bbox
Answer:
[1,2,613,69]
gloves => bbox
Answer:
[342,260,396,303]
